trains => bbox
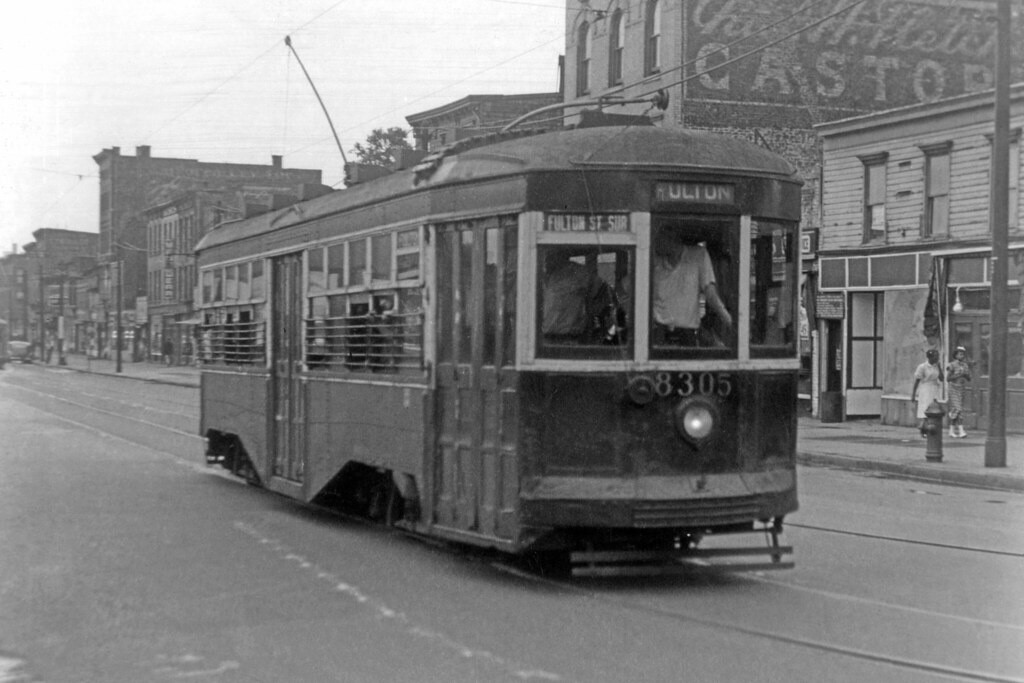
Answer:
[190,112,805,577]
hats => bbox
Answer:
[957,347,966,352]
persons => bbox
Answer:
[945,346,972,438]
[542,251,603,345]
[911,349,944,438]
[654,227,734,346]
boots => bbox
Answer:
[959,425,967,438]
[949,425,959,437]
